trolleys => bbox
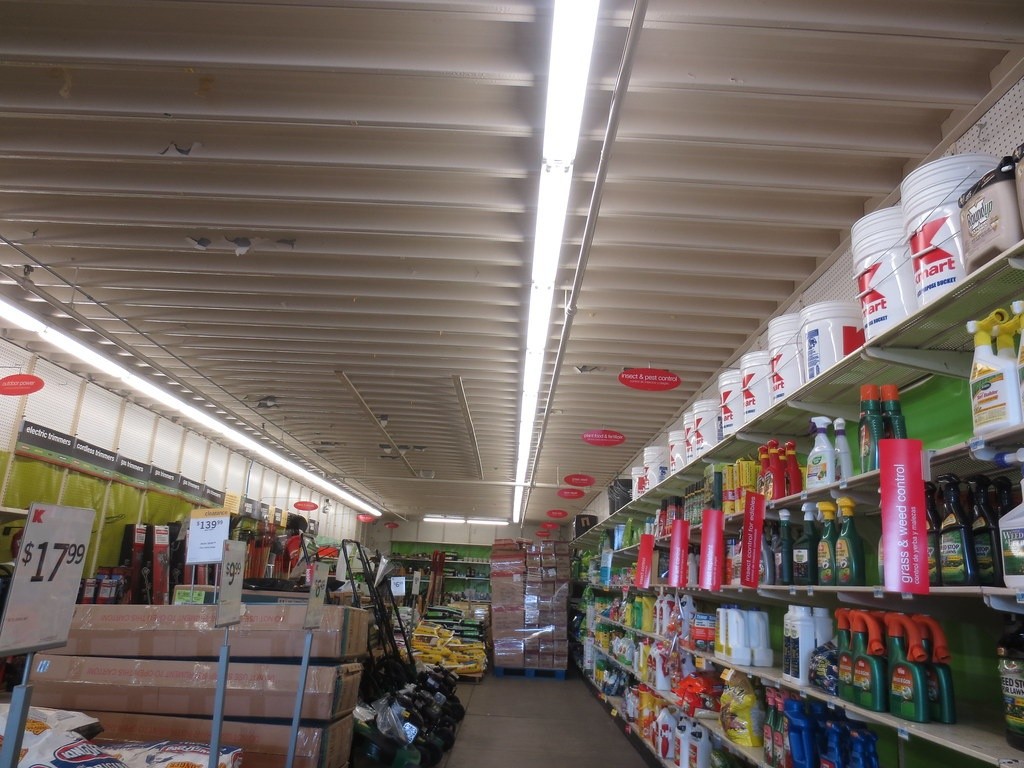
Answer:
[341,538,466,768]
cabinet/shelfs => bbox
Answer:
[386,557,490,603]
[566,237,1023,768]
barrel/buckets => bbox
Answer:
[608,144,1024,515]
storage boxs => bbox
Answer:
[489,538,571,671]
[24,585,368,768]
